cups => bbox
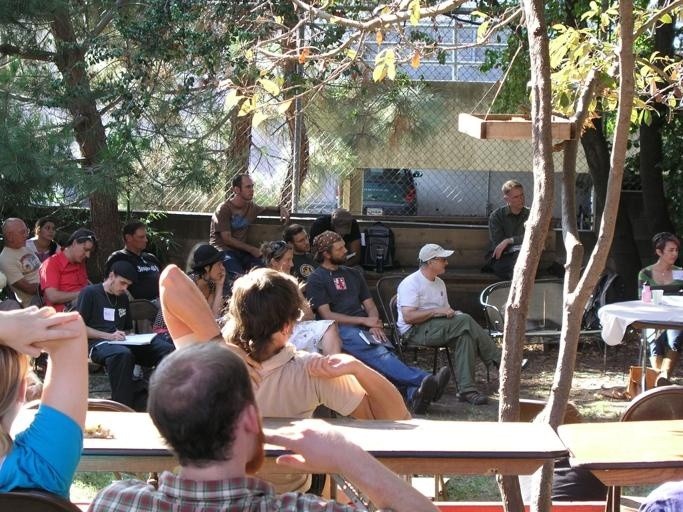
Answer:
[652,290,665,305]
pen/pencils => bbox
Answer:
[113,324,126,340]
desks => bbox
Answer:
[597,292,682,401]
[554,418,682,512]
[15,408,569,512]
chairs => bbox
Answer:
[519,396,584,428]
[127,298,157,336]
[373,269,463,397]
[24,396,160,496]
[604,383,682,512]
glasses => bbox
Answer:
[272,241,286,258]
[78,236,95,242]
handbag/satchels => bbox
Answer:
[627,365,660,400]
[362,222,394,269]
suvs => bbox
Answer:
[333,167,423,218]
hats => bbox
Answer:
[418,243,454,261]
[191,244,226,269]
[331,210,353,236]
[109,261,138,283]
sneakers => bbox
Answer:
[458,392,487,405]
[522,359,528,369]
[657,376,682,386]
[433,366,452,402]
[132,364,144,380]
[412,374,437,414]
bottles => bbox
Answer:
[642,280,652,303]
[376,249,385,273]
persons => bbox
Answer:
[639,480,682,511]
[482,179,565,282]
[638,232,682,388]
[518,401,608,502]
[397,243,528,406]
[1,175,452,511]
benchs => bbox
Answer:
[201,221,558,334]
[476,274,609,376]
